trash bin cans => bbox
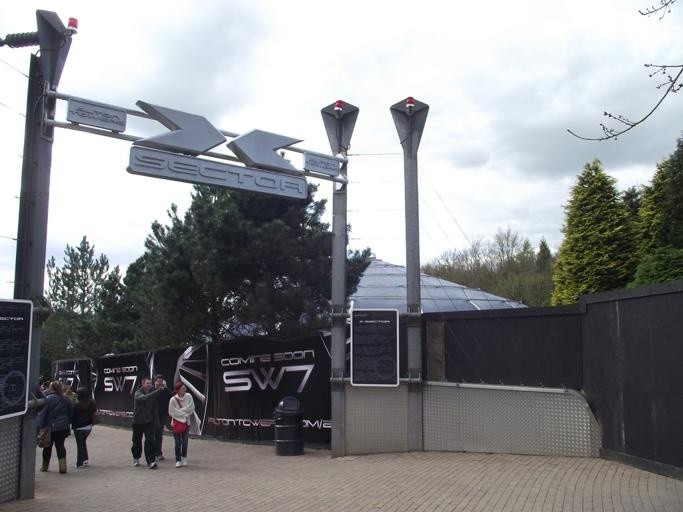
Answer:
[273,396,305,455]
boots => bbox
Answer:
[38,457,67,474]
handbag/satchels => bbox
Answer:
[36,426,53,449]
[172,418,189,433]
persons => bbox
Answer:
[34,375,98,473]
[149,374,172,461]
[167,380,195,468]
[129,376,165,469]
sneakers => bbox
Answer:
[74,459,89,469]
[174,455,189,468]
[131,455,166,470]
